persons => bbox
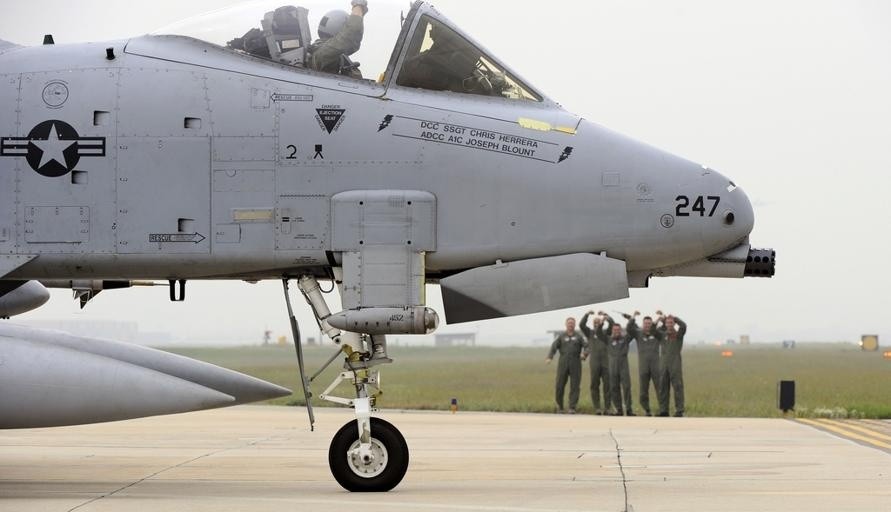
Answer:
[627,310,670,416]
[580,311,617,416]
[650,314,688,417]
[311,1,370,80]
[595,312,639,416]
[547,317,592,416]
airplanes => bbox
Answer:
[2,1,775,493]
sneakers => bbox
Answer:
[646,409,683,417]
[558,408,576,414]
[595,408,635,415]
